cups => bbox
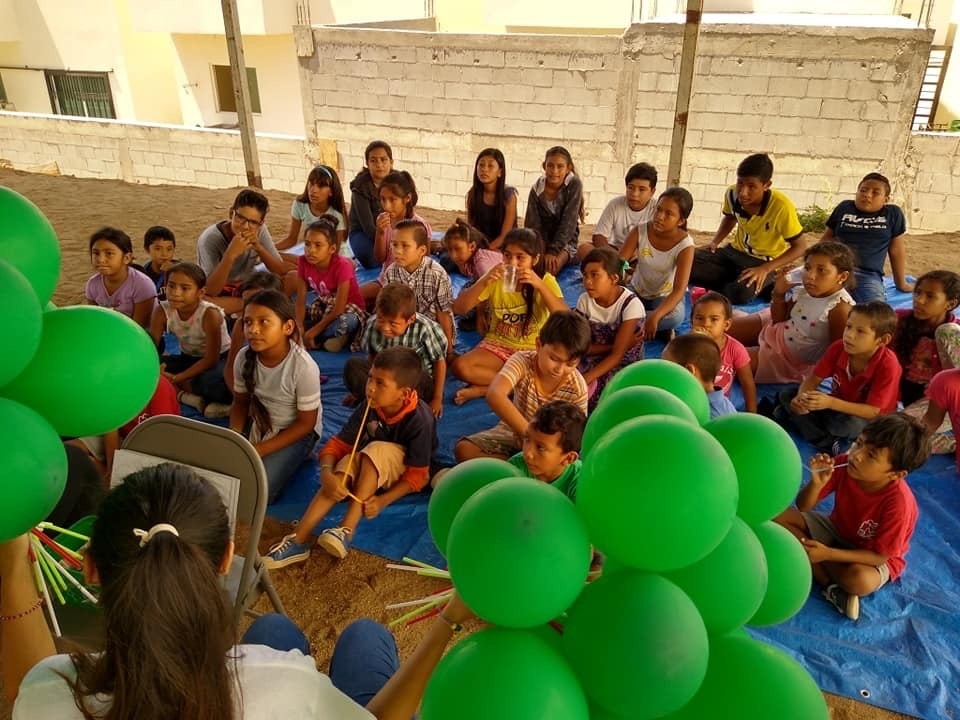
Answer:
[503,265,520,293]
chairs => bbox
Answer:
[50,414,285,650]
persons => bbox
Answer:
[203,271,309,420]
[498,398,603,583]
[144,226,181,293]
[785,412,925,627]
[0,460,473,720]
[38,307,182,543]
[261,346,435,569]
[197,140,960,476]
[228,289,324,506]
[148,263,232,411]
[83,227,159,340]
[342,282,448,420]
[453,311,592,462]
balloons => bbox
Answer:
[425,359,829,720]
[0,184,162,539]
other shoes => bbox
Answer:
[317,526,352,559]
[204,403,232,419]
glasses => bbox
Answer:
[235,209,264,226]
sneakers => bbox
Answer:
[260,533,310,569]
[821,580,859,621]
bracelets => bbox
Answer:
[0,591,45,621]
[434,610,466,634]
